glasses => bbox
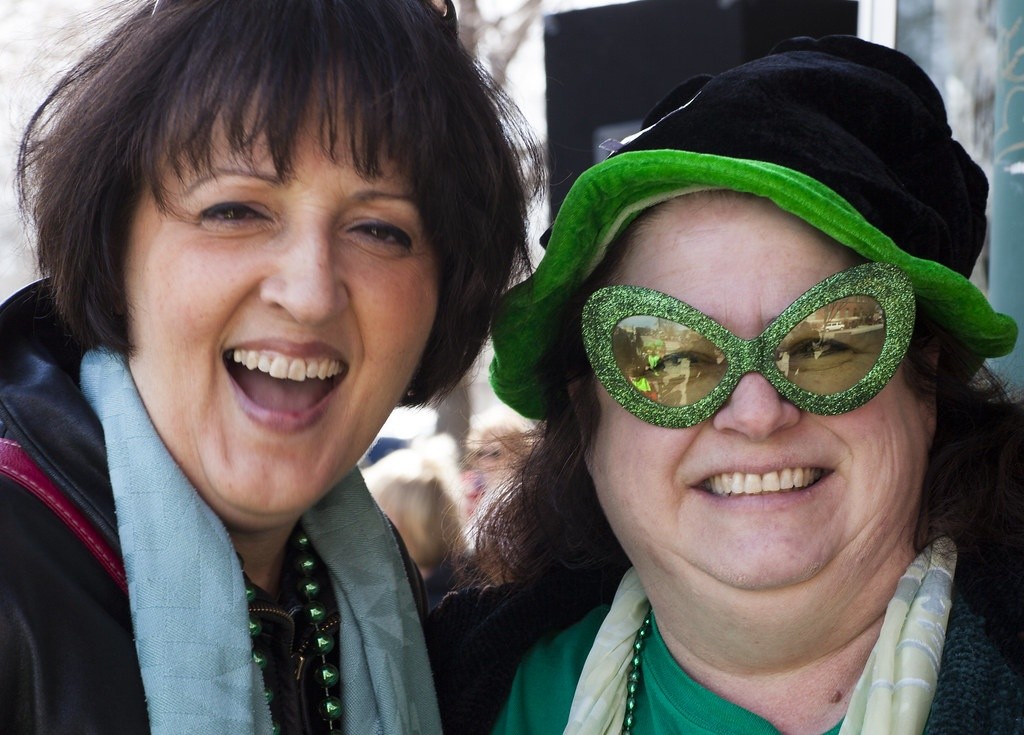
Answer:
[581,261,916,428]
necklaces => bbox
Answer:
[625,612,653,733]
[233,533,339,732]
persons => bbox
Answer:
[467,30,1023,735]
[367,405,537,584]
[0,0,534,734]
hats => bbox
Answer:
[487,34,1018,421]
